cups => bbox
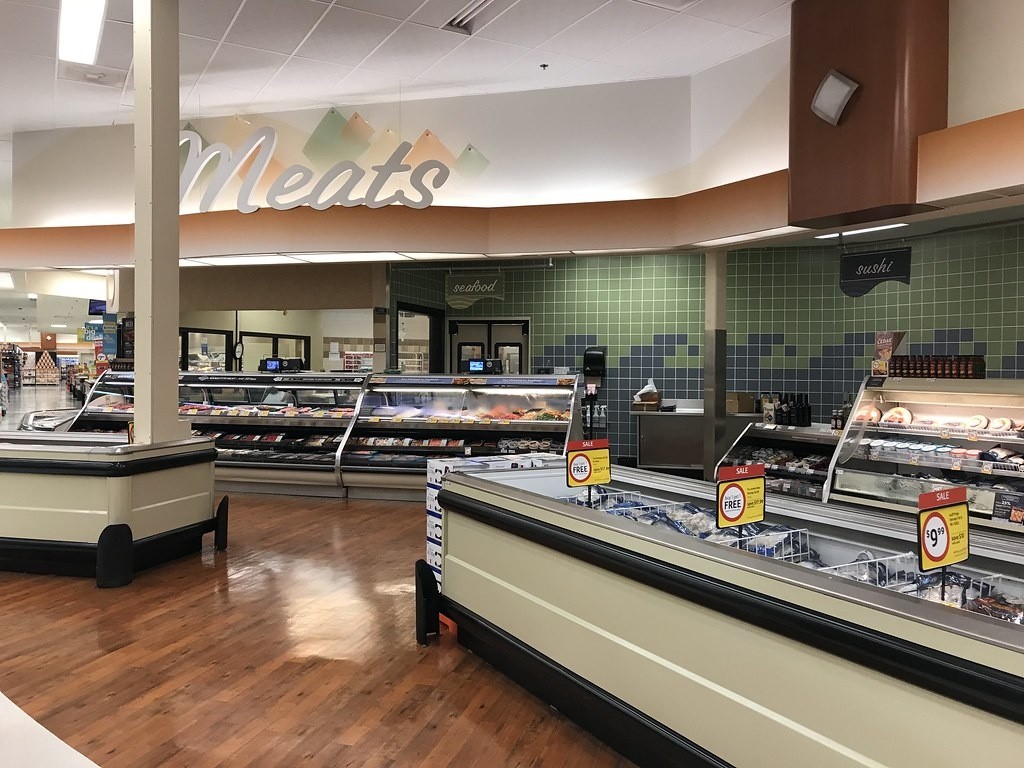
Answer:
[843,435,984,467]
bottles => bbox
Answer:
[759,392,782,413]
[836,409,844,430]
[830,408,837,429]
[775,393,814,427]
[889,354,986,379]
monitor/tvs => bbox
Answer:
[88,299,106,315]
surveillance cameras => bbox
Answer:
[28,293,37,301]
